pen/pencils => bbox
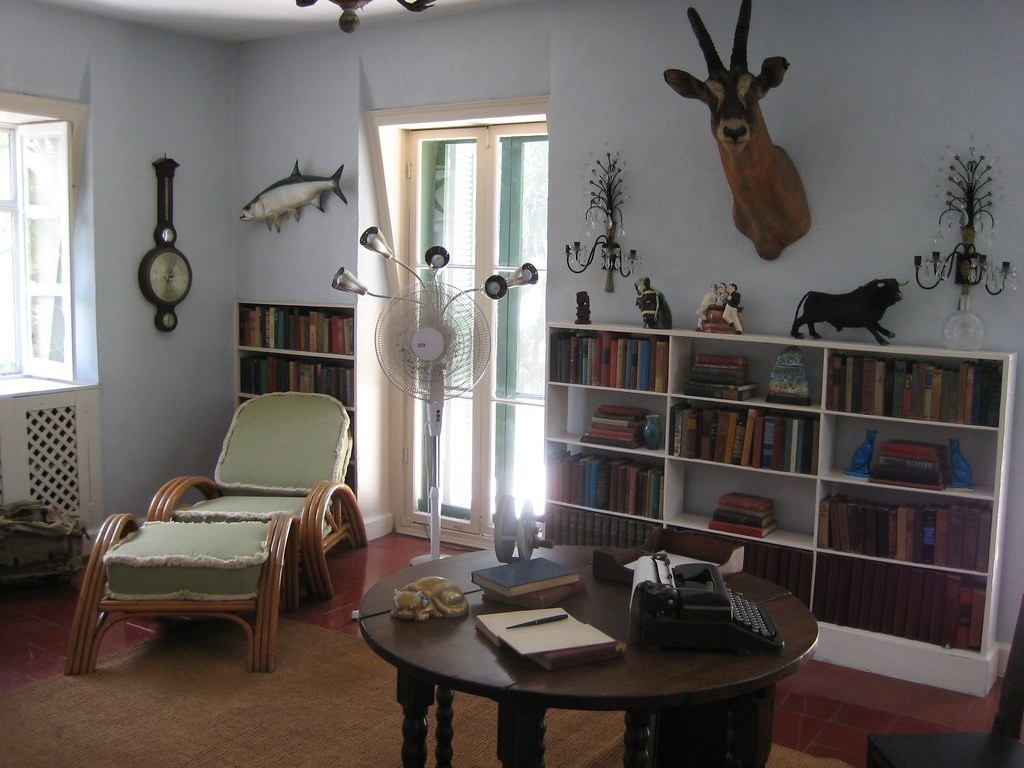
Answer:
[506,613,568,629]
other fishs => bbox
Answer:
[239,158,349,232]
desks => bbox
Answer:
[358,544,819,768]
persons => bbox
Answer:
[634,276,745,336]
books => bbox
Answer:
[666,352,821,480]
[474,608,627,673]
[813,551,988,655]
[544,503,663,553]
[707,491,779,539]
[824,354,1004,428]
[868,438,954,493]
[238,305,356,405]
[470,556,586,610]
[548,330,669,394]
[579,404,647,449]
[545,449,665,523]
[817,492,993,573]
[742,538,813,610]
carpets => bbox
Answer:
[0,613,857,768]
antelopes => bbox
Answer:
[660,0,814,261]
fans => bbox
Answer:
[375,281,491,565]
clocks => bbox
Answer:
[139,152,192,333]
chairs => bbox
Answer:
[869,593,1024,768]
[63,393,368,674]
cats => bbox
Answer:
[390,577,470,621]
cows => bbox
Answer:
[790,276,910,347]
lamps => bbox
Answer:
[331,227,539,318]
[914,136,1019,312]
[563,150,643,293]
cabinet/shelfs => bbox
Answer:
[543,321,1017,700]
[235,300,357,508]
